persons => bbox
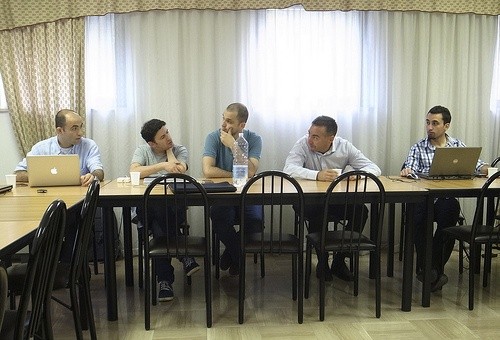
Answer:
[283,115,381,282]
[201,103,262,276]
[15,109,104,288]
[130,119,200,302]
[400,106,492,292]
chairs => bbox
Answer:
[137,167,388,330]
[1,199,66,340]
[400,157,500,311]
[8,178,100,340]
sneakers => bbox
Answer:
[182,256,200,276]
[158,280,174,301]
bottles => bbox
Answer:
[232,133,249,188]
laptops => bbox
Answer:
[0,184,13,194]
[416,147,482,180]
[168,182,238,194]
[27,155,81,187]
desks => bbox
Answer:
[0,177,500,340]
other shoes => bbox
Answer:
[331,254,353,281]
[317,260,333,281]
[417,267,438,285]
[220,249,231,270]
[230,257,242,274]
[431,274,448,292]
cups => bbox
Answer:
[6,174,17,191]
[332,169,342,176]
[130,172,140,185]
[488,168,498,178]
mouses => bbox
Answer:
[407,173,420,180]
